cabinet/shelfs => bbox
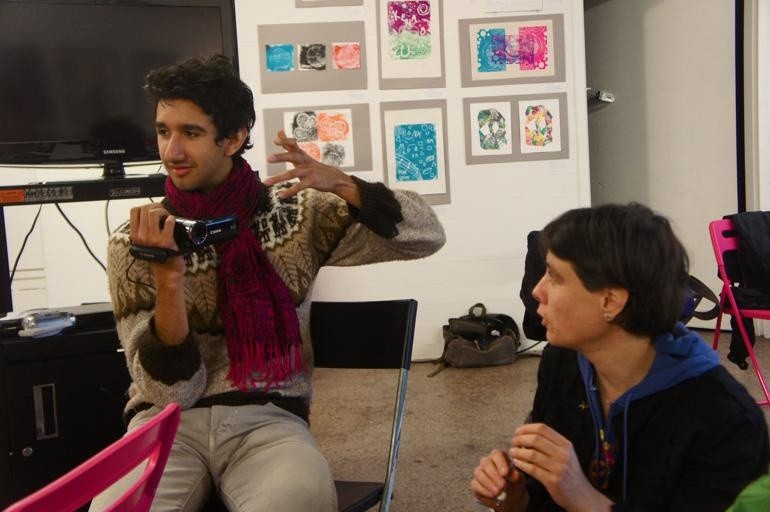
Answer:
[0,303,130,486]
[0,352,129,510]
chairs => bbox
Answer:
[708,218,770,406]
[5,402,181,511]
[309,299,418,512]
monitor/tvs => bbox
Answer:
[0,0,240,177]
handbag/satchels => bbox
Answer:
[444,303,519,367]
[684,273,720,320]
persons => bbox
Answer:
[468,201,769,511]
[86,54,447,512]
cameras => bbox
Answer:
[158,212,240,250]
